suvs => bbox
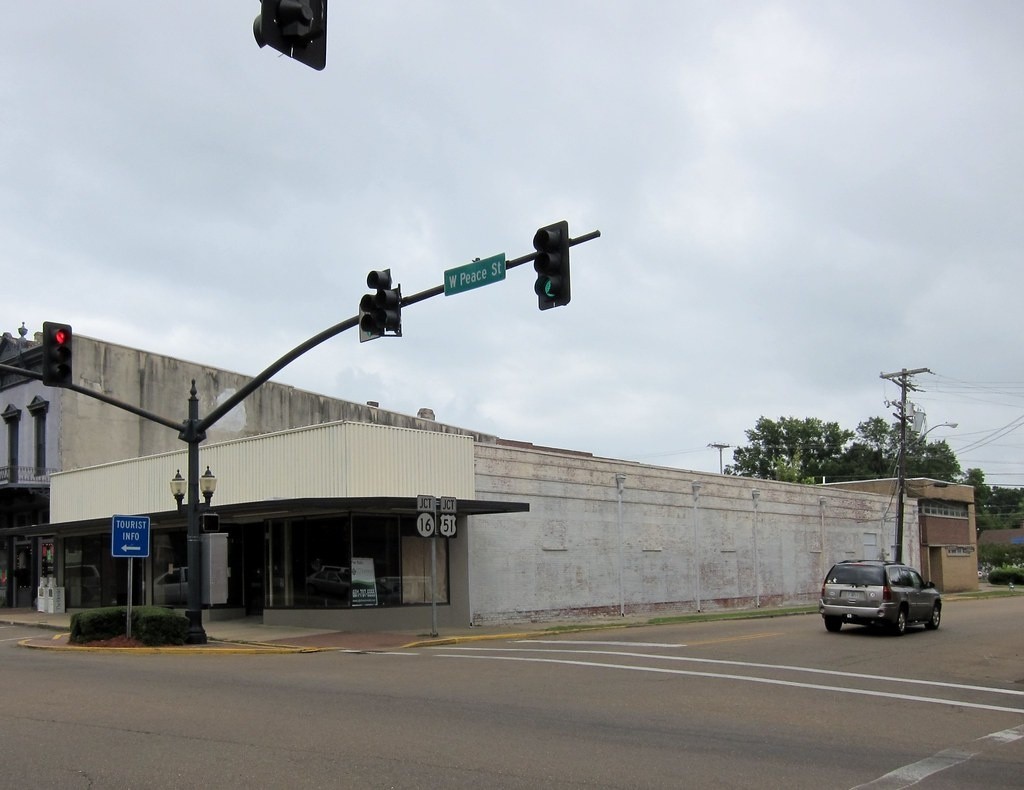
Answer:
[819,558,943,635]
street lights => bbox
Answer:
[169,464,218,642]
[890,423,960,561]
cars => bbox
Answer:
[309,566,354,601]
[61,565,99,603]
[152,564,191,605]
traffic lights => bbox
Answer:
[358,268,407,335]
[532,220,572,310]
[42,321,75,389]
